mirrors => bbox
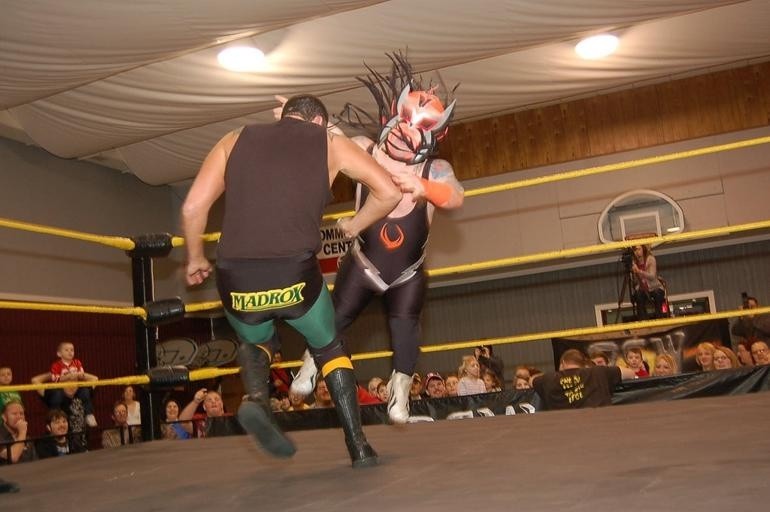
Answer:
[597,189,686,244]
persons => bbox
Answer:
[181,93,404,469]
[1,343,98,465]
[269,349,335,413]
[160,388,225,439]
[629,245,665,321]
[354,339,544,404]
[102,385,142,450]
[290,44,465,426]
[529,297,769,411]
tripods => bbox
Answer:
[614,267,661,321]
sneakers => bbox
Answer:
[85,413,98,427]
[290,347,322,396]
[385,369,415,423]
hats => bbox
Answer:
[424,372,444,388]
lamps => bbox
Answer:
[217,36,267,73]
[575,34,619,61]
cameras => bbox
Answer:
[620,248,634,263]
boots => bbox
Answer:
[236,341,295,456]
[323,367,379,468]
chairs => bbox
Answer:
[633,277,669,318]
